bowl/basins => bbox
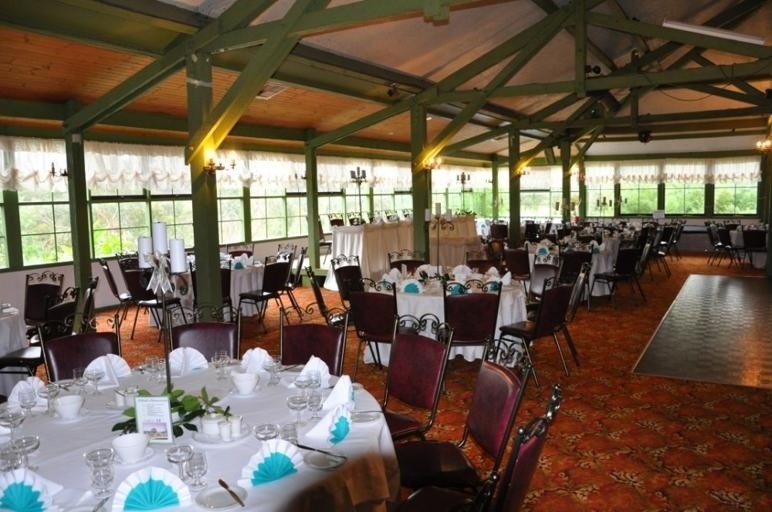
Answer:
[51,370,260,461]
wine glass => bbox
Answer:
[0,351,323,502]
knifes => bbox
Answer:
[49,380,68,391]
[218,479,244,507]
[92,496,109,512]
[296,443,349,460]
[282,363,301,372]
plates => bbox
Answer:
[55,385,259,466]
[350,411,380,422]
[63,504,109,512]
[352,382,365,390]
[304,451,345,470]
[195,484,249,509]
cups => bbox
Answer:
[115,389,138,408]
[203,415,244,441]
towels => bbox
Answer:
[0,468,60,512]
[237,438,305,488]
[112,466,193,512]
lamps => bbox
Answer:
[204,150,237,175]
[753,114,772,155]
[661,18,765,46]
[421,158,442,175]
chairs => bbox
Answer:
[398,383,563,511]
[394,336,532,490]
[704,219,771,273]
